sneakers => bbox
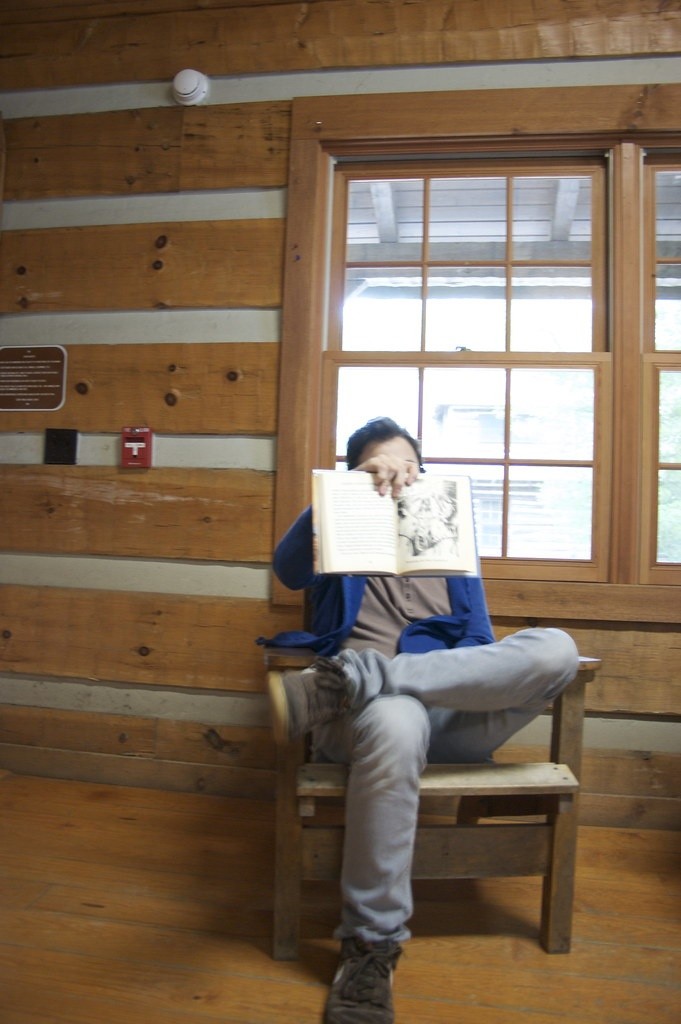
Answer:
[324,936,403,1024]
[266,657,351,745]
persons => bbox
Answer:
[266,419,581,1023]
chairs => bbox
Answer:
[260,645,601,961]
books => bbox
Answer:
[313,471,479,578]
